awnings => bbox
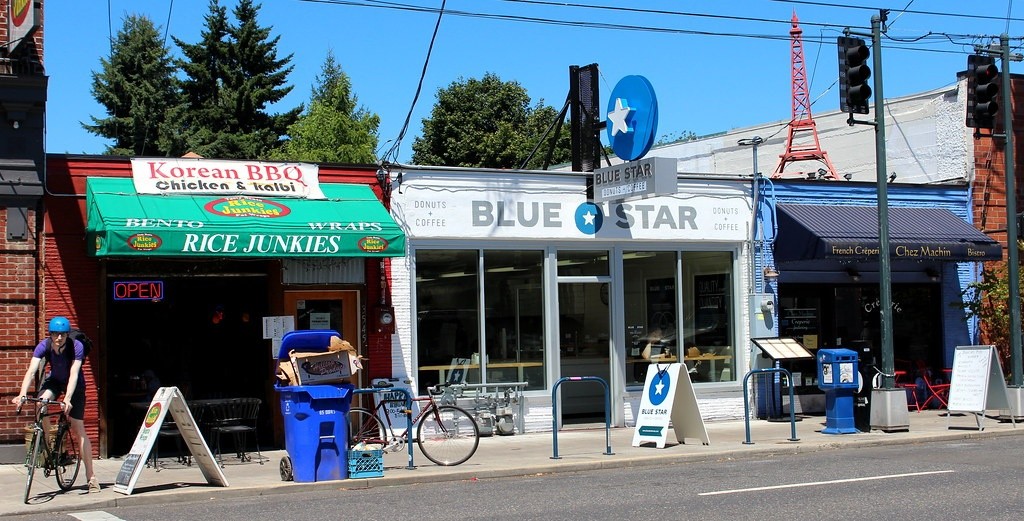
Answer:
[87,177,405,257]
[776,203,1003,263]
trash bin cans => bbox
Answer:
[273,328,354,482]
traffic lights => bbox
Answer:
[837,36,872,114]
[966,55,998,129]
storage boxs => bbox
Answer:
[348,449,385,480]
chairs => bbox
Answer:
[894,371,920,413]
[138,398,262,472]
[917,358,950,411]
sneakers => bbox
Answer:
[87,475,100,493]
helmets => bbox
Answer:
[48,317,71,332]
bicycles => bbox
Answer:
[347,380,480,466]
[15,395,82,504]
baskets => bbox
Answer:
[348,449,384,478]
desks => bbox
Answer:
[631,355,732,382]
[131,398,262,466]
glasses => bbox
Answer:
[50,331,69,337]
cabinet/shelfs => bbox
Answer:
[785,308,817,338]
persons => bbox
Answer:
[12,316,100,494]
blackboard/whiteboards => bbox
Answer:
[946,345,994,414]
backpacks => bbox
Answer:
[45,329,94,371]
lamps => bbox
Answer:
[765,265,779,282]
[845,267,862,281]
[807,172,816,180]
[537,259,588,265]
[441,271,476,278]
[486,267,527,272]
[889,172,897,183]
[845,174,852,181]
[597,252,656,260]
[818,168,827,180]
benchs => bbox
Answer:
[696,346,732,381]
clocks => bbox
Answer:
[600,283,609,304]
